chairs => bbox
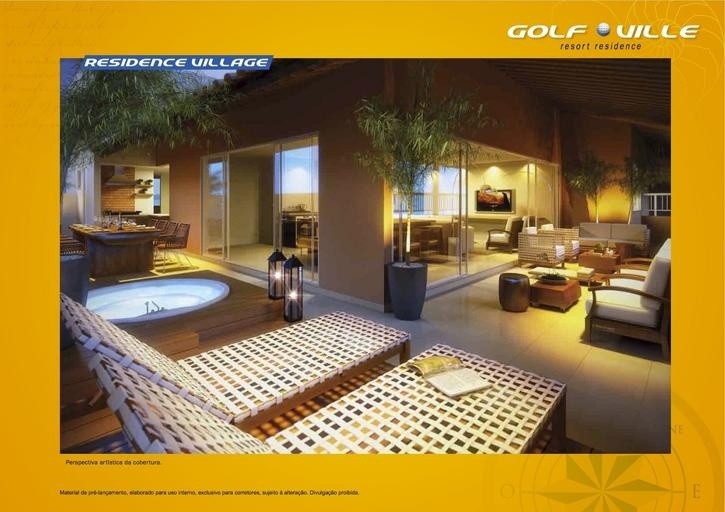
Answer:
[516,224,580,271]
[585,236,669,359]
[485,216,523,253]
[146,218,192,273]
[60,292,566,455]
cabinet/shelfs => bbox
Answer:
[106,182,154,197]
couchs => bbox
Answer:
[573,222,650,255]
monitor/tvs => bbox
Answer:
[477,190,512,212]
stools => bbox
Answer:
[498,273,530,313]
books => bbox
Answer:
[408,356,491,396]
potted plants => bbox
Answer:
[352,61,500,324]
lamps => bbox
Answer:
[268,247,305,322]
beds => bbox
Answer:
[68,224,160,279]
